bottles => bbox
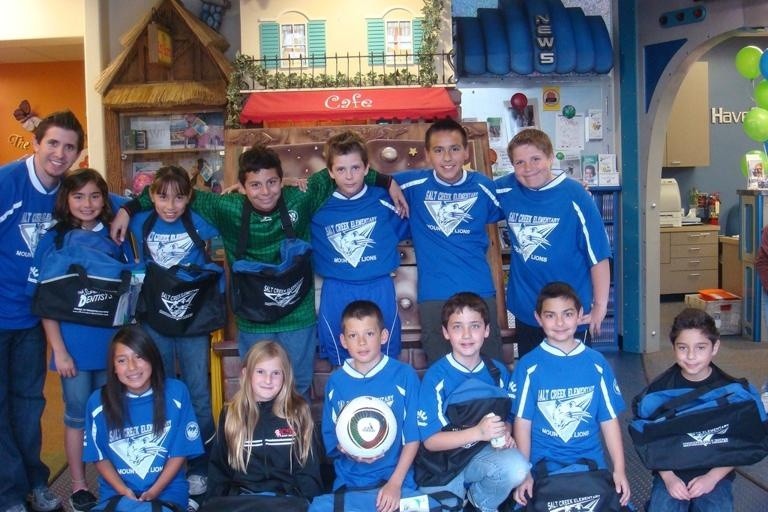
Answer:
[689,187,699,208]
[486,410,507,449]
[708,196,720,223]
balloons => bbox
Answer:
[562,104,576,120]
[753,79,768,110]
[511,93,527,110]
[488,149,497,164]
[556,151,565,159]
[735,46,763,80]
[741,150,768,180]
[759,47,768,81]
[742,107,768,143]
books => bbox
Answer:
[589,190,614,342]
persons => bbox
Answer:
[585,165,597,181]
[107,141,413,416]
[210,339,323,511]
[320,300,422,511]
[219,132,413,365]
[81,324,206,512]
[507,280,639,510]
[295,115,513,365]
[487,129,613,356]
[417,291,533,511]
[94,165,310,498]
[0,110,84,511]
[631,307,741,511]
[504,232,512,246]
[27,167,135,511]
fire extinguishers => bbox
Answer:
[708,191,721,225]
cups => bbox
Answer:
[126,129,136,150]
[136,129,147,150]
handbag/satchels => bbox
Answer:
[411,377,511,485]
[91,485,462,512]
[628,378,767,470]
[506,458,637,511]
[32,229,314,336]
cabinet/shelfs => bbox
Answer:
[719,236,744,299]
[659,225,721,303]
[662,60,710,168]
[118,107,225,199]
[736,190,768,343]
[496,186,620,352]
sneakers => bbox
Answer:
[185,474,210,495]
[5,483,97,512]
